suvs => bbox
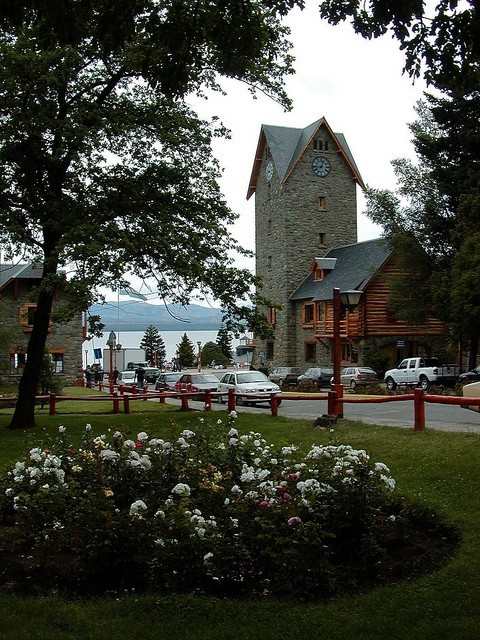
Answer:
[297,368,334,387]
[270,367,303,386]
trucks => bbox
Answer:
[103,348,160,384]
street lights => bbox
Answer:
[197,341,201,371]
[110,343,122,394]
[331,287,364,418]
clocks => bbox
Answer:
[311,156,331,179]
[265,162,274,181]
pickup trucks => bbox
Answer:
[383,356,459,390]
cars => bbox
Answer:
[220,370,281,406]
[116,371,138,385]
[330,367,379,389]
[155,373,184,391]
[458,364,480,384]
[175,373,220,401]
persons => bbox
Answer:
[147,357,178,373]
[95,366,105,390]
[85,365,93,384]
[258,363,269,377]
[248,365,257,371]
[113,367,120,385]
[135,366,146,394]
